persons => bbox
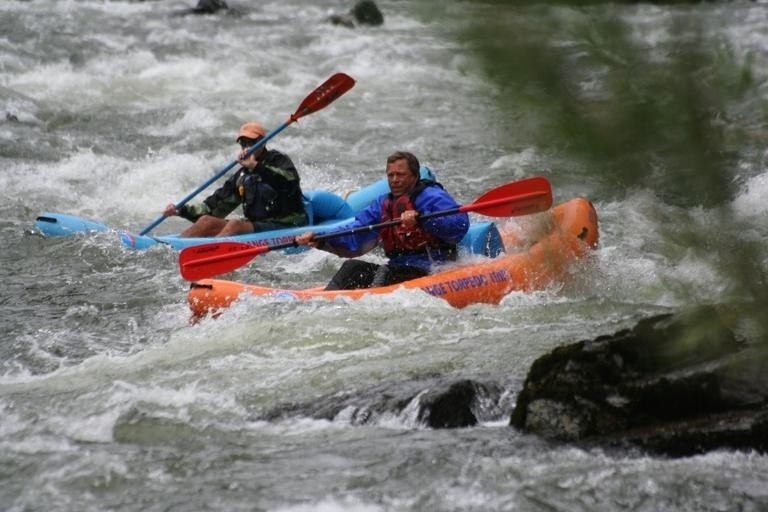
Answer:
[294,150,472,293]
[162,123,311,239]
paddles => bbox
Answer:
[179,176,553,281]
[139,72,355,237]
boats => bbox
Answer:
[36,160,438,255]
[186,196,603,325]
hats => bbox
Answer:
[236,123,264,142]
